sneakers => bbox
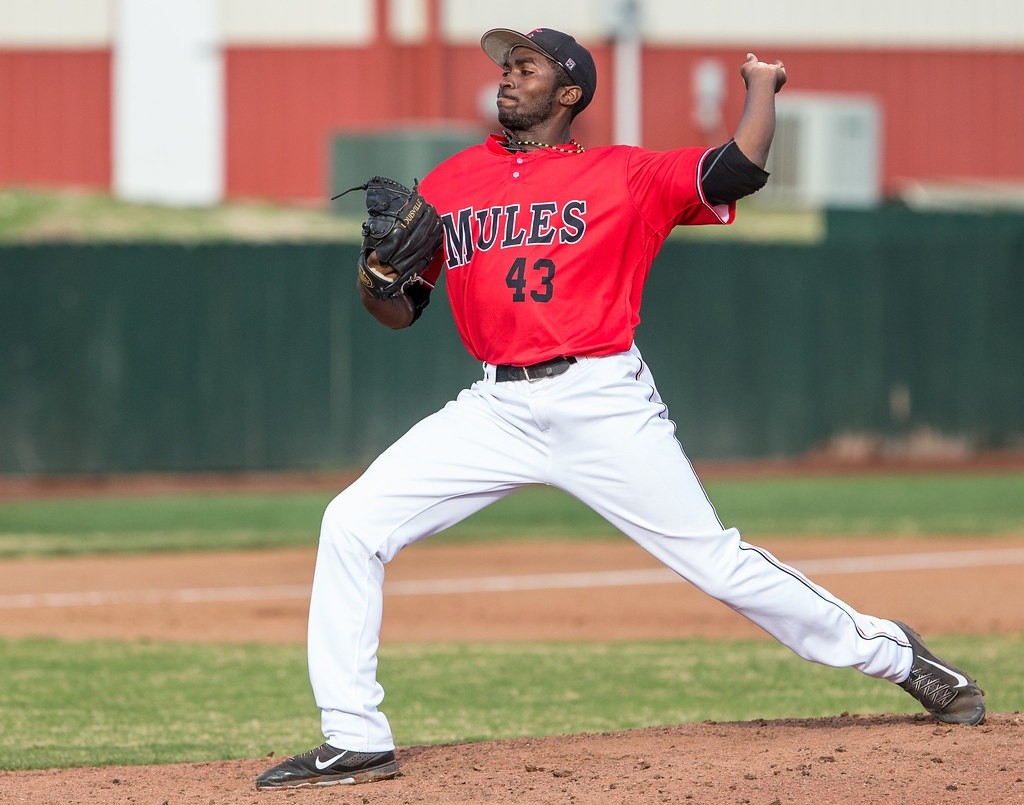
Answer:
[888,620,986,727]
[256,744,400,790]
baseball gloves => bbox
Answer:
[356,175,443,300]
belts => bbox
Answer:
[485,356,578,383]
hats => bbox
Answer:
[481,28,597,99]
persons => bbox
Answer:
[257,28,985,790]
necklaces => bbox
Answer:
[501,130,584,153]
